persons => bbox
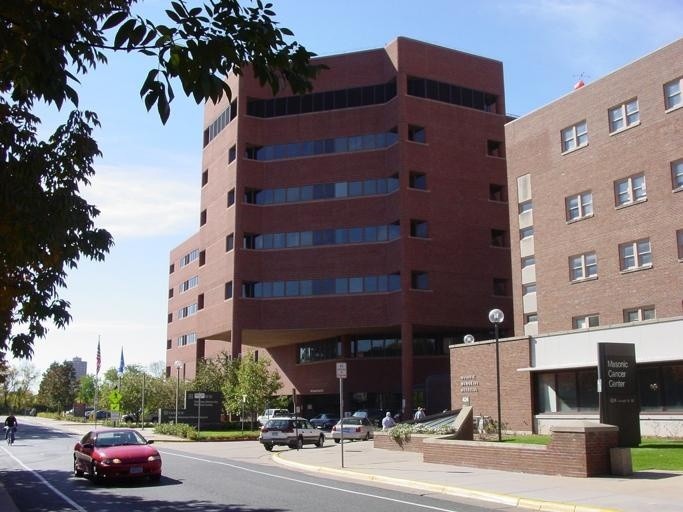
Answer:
[443,408,448,413]
[382,411,396,429]
[5,413,17,443]
[414,407,425,421]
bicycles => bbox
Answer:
[4,426,16,446]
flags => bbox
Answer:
[96,339,101,374]
[117,347,124,379]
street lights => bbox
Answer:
[116,371,124,393]
[488,307,505,442]
[174,359,184,425]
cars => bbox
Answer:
[330,416,374,443]
[259,416,326,451]
[85,407,111,419]
[72,428,162,485]
[256,408,289,426]
[351,411,369,418]
[309,413,337,429]
